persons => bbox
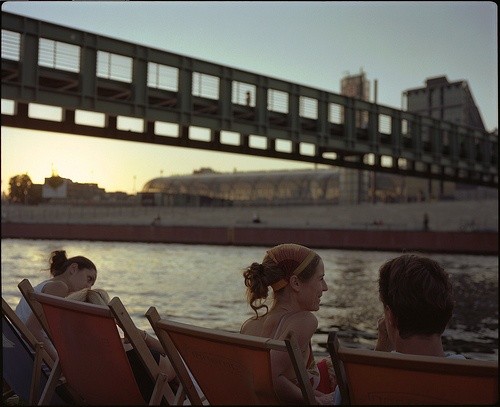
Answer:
[334,255,488,407]
[10,249,180,407]
[233,243,336,407]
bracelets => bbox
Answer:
[144,330,148,340]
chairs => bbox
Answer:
[145,307,319,406]
[325,331,499,406]
[18,279,186,406]
[0,296,85,406]
